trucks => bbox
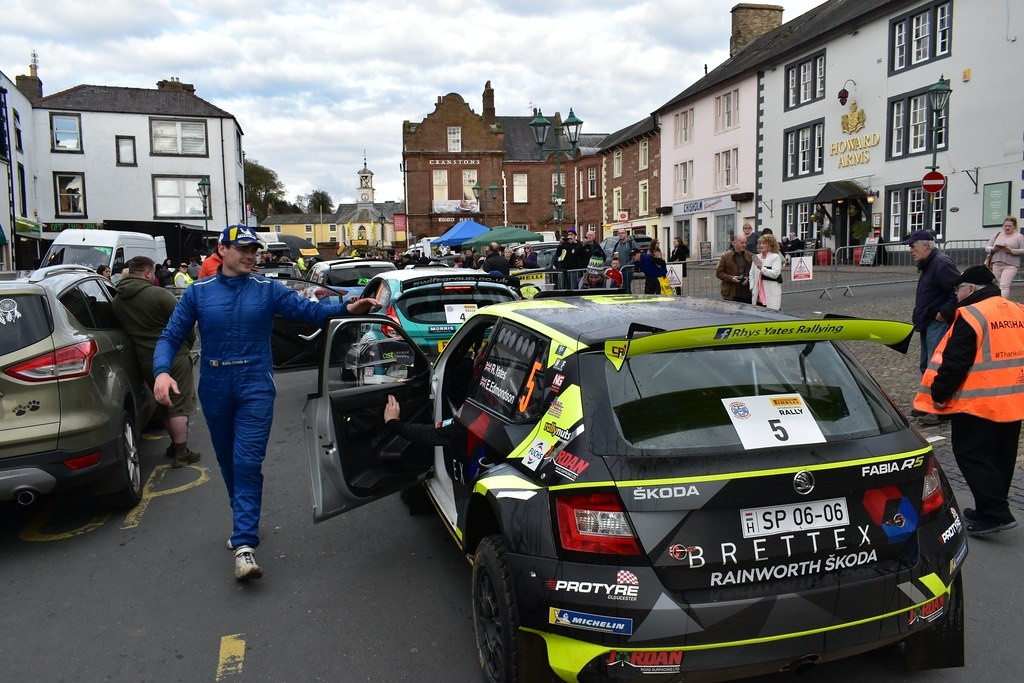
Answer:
[404,236,460,261]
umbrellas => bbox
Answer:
[428,220,490,248]
[462,226,544,248]
[212,230,324,264]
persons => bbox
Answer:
[151,225,382,581]
[366,239,553,277]
[112,256,202,469]
[985,217,1024,299]
[901,229,960,426]
[552,227,691,295]
[714,222,805,311]
[384,350,506,484]
[912,266,1024,534]
[95,251,317,328]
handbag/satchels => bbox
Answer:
[985,232,1000,270]
[657,276,674,296]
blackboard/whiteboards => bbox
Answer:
[699,241,711,260]
[858,236,887,265]
[802,238,821,256]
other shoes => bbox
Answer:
[165,444,200,468]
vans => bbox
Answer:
[303,255,399,306]
[39,228,168,286]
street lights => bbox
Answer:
[197,175,211,248]
[378,213,386,248]
[528,106,584,241]
[472,180,500,227]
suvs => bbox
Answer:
[255,262,303,281]
[0,263,161,513]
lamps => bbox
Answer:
[838,79,856,106]
[867,190,879,203]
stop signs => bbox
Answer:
[921,172,947,193]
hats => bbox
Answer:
[952,264,996,287]
[587,256,605,276]
[901,229,934,246]
[629,247,640,257]
[218,225,264,249]
[566,228,577,235]
[178,262,189,268]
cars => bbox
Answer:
[263,268,970,683]
[510,242,560,268]
[599,234,663,265]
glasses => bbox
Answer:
[524,247,530,250]
[954,283,970,292]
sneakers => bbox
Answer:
[963,508,978,519]
[227,537,233,549]
[966,514,1017,534]
[911,409,929,416]
[234,545,263,579]
[917,413,948,425]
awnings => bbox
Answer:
[811,179,867,204]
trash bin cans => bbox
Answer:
[854,248,864,266]
[818,248,830,265]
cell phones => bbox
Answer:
[752,255,762,264]
[563,238,569,241]
